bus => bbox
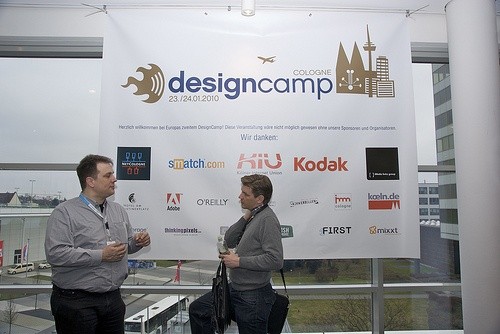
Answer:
[124,296,189,334]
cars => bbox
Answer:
[7,262,34,275]
[38,263,51,269]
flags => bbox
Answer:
[173,262,181,284]
[23,242,28,260]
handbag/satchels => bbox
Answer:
[212,260,231,334]
[267,294,289,334]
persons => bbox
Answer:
[189,174,284,334]
[45,155,151,334]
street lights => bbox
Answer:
[30,180,36,203]
[58,192,62,203]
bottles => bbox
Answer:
[217,236,230,256]
